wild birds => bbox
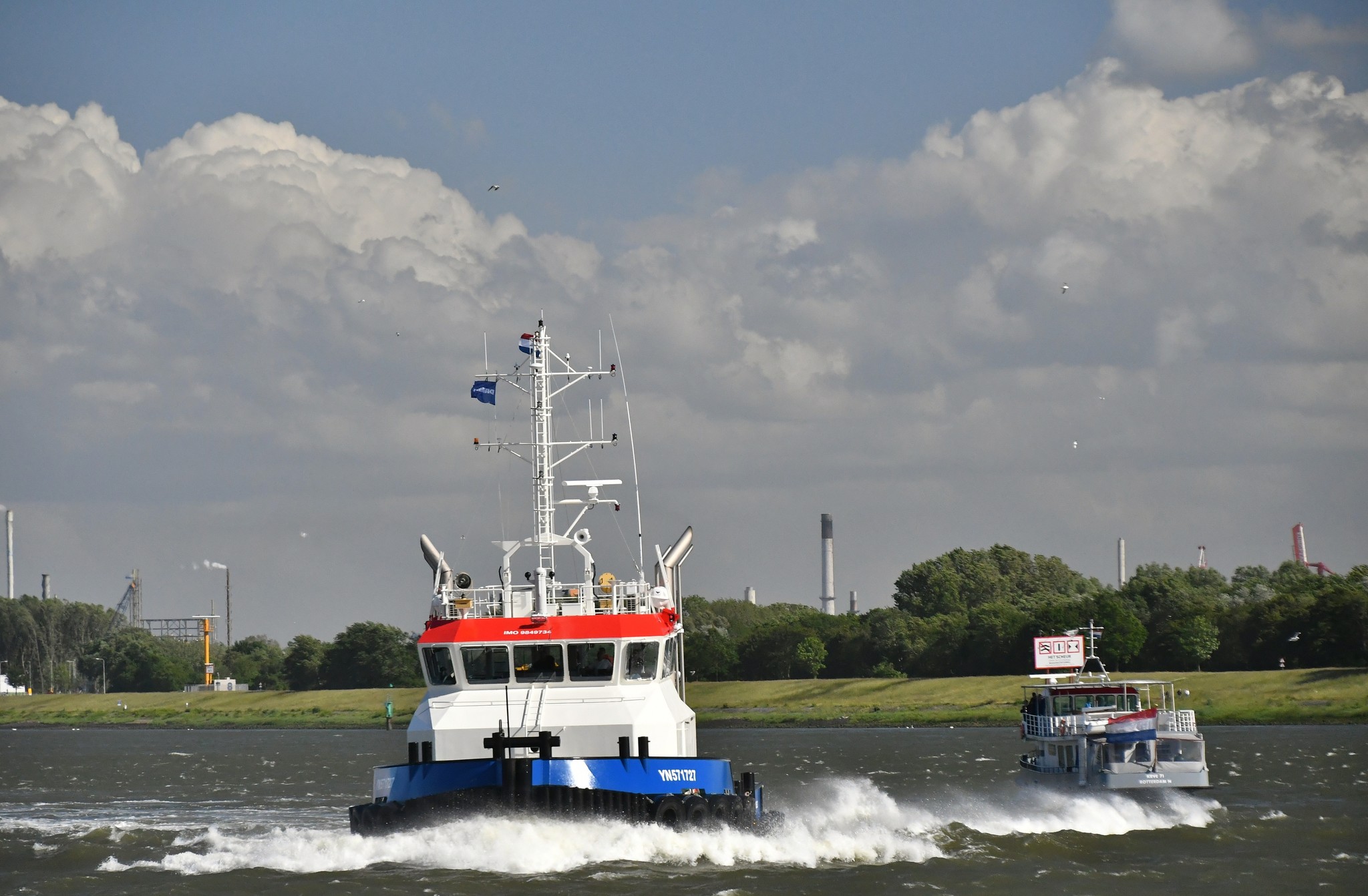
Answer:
[487,185,500,191]
[1288,632,1301,642]
[1060,282,1070,294]
[358,299,365,303]
[911,725,914,729]
[1072,440,1078,449]
[395,332,401,337]
[1099,397,1105,401]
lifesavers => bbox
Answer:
[652,793,756,831]
[1060,719,1066,736]
[360,803,385,835]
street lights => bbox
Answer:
[49,660,53,689]
[96,658,106,694]
[28,661,32,688]
[66,659,76,692]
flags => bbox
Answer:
[470,380,497,405]
[1105,708,1157,744]
[518,332,542,358]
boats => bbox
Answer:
[1017,618,1218,796]
[349,309,789,842]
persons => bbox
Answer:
[628,649,643,675]
[579,647,613,676]
[1027,692,1037,715]
[1177,688,1182,699]
[1185,689,1190,696]
